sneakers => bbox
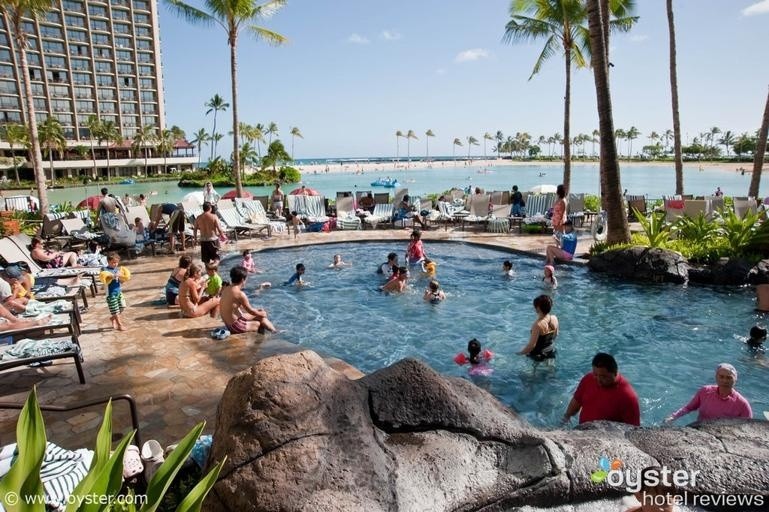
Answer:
[211,327,228,337]
[217,329,230,339]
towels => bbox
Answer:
[0,434,213,512]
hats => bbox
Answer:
[562,220,572,226]
[4,266,26,282]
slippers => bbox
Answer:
[122,445,144,478]
[141,439,166,483]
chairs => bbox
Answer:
[101,188,768,260]
[0,193,109,390]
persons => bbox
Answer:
[715,187,723,195]
[745,324,768,352]
[0,181,579,336]
[291,157,498,181]
[454,339,493,370]
[665,362,754,424]
[558,353,641,427]
[516,295,559,368]
[622,466,681,512]
